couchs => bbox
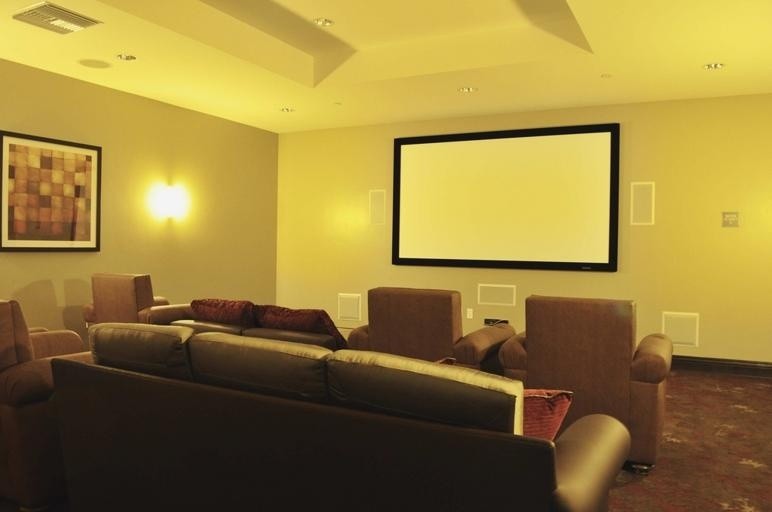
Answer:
[48,357,632,512]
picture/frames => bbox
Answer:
[0,131,101,252]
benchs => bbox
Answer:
[171,321,339,352]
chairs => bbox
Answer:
[83,270,191,324]
[0,300,95,512]
[499,295,673,475]
[348,287,515,374]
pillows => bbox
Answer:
[255,305,346,349]
[190,299,252,327]
